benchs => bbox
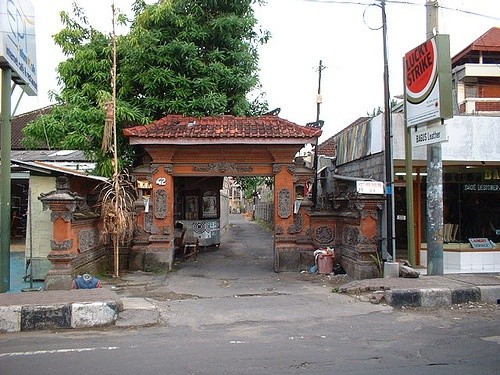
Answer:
[181,237,198,261]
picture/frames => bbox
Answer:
[182,190,220,220]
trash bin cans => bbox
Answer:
[313,246,335,274]
